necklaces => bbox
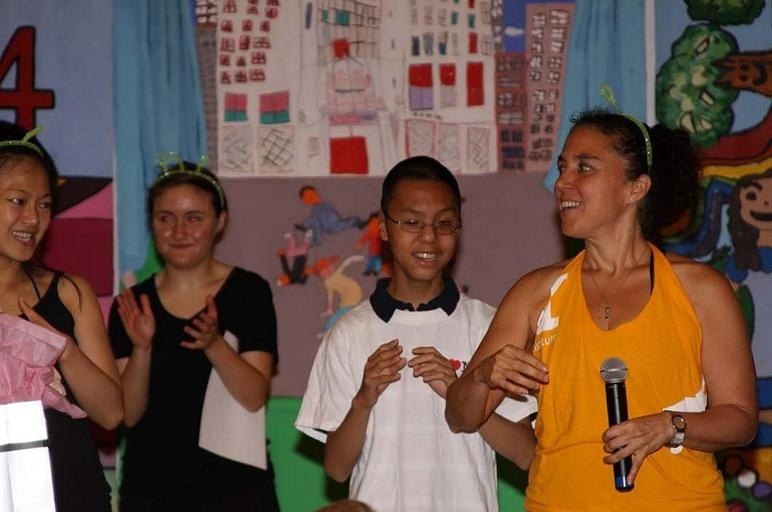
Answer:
[586,244,652,320]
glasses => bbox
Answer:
[384,213,460,234]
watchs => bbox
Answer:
[665,409,686,449]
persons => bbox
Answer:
[292,149,536,512]
[109,164,281,511]
[444,107,759,512]
[276,188,386,337]
[0,127,123,511]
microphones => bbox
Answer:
[599,357,639,492]
